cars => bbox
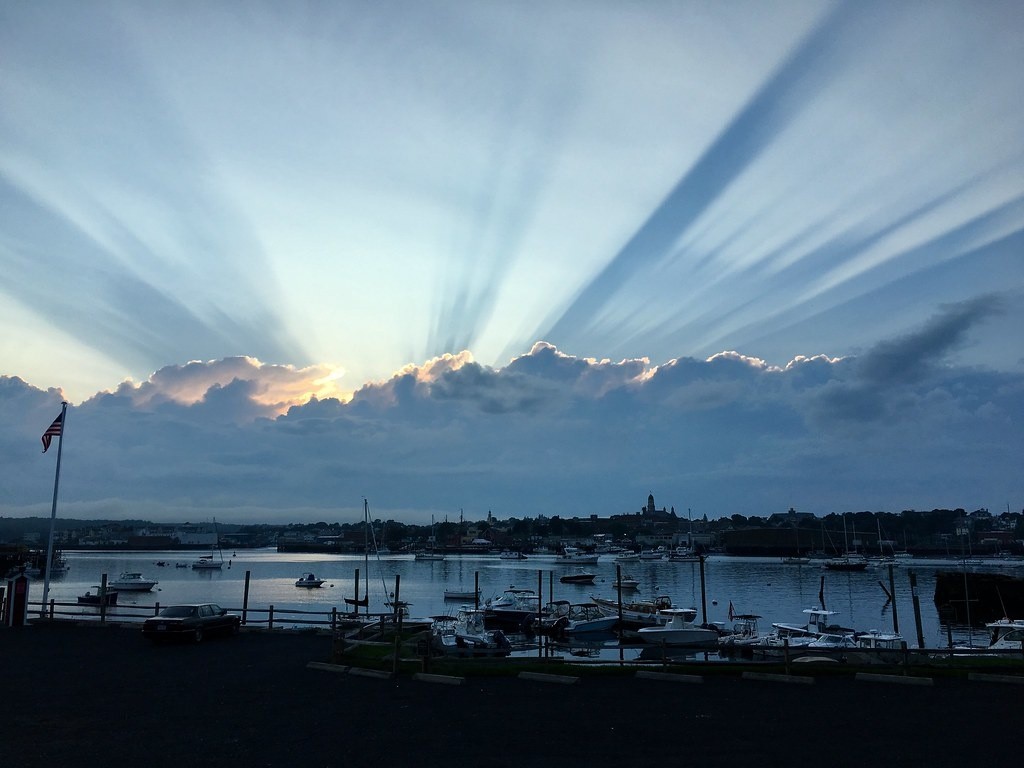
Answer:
[143,602,242,643]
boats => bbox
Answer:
[554,547,600,565]
[77,585,118,606]
[612,577,640,588]
[785,557,812,565]
[559,567,597,583]
[415,554,445,561]
[295,579,327,587]
[499,551,528,560]
[825,551,870,573]
[662,550,710,562]
[614,550,641,563]
[444,587,483,600]
[107,572,159,592]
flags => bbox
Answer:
[728,603,735,622]
[42,410,63,452]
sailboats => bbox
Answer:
[326,554,1024,657]
[191,515,226,569]
